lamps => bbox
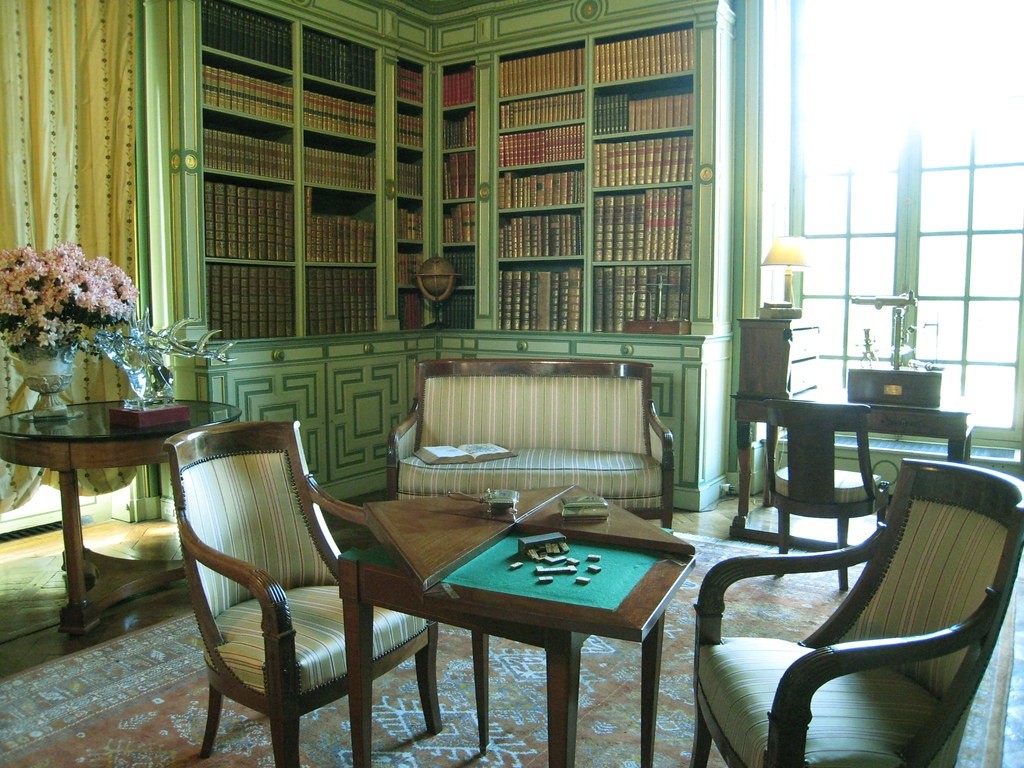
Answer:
[759,236,813,307]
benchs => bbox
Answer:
[383,358,675,529]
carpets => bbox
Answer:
[0,530,1016,768]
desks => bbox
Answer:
[338,484,696,768]
[0,400,243,638]
[730,389,977,528]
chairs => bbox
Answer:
[689,456,1024,768]
[162,419,443,768]
[762,399,890,592]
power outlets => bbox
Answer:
[719,478,728,497]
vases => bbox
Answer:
[18,346,77,417]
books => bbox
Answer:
[413,441,516,465]
[759,301,802,319]
[203,0,693,340]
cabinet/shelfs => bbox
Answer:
[166,0,737,513]
[736,318,821,400]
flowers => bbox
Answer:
[0,240,140,354]
